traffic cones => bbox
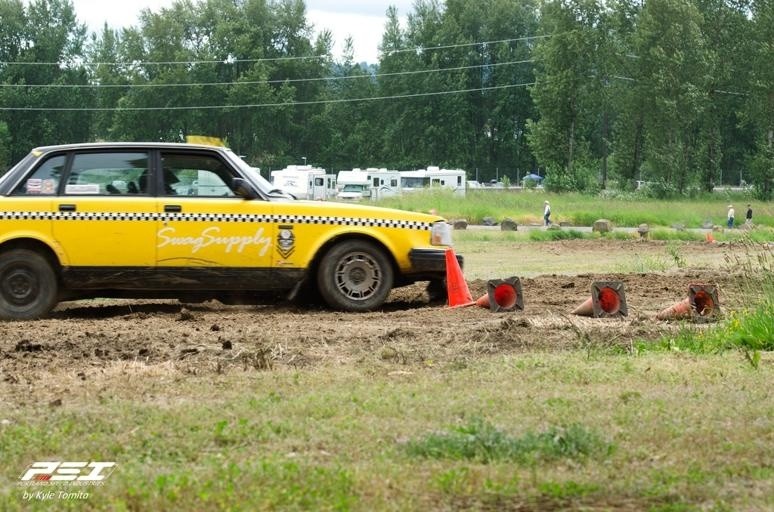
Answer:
[475,275,526,313]
[572,280,630,320]
[657,285,723,325]
[442,247,480,311]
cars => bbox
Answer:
[337,185,371,202]
[1,142,463,323]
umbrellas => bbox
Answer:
[522,174,543,180]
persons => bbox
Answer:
[745,203,752,230]
[544,200,552,226]
[727,205,734,229]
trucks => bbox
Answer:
[195,154,470,205]
